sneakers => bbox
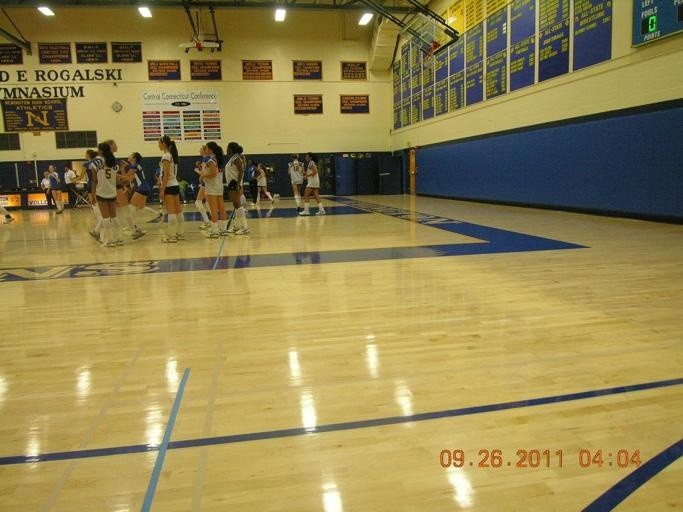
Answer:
[102,239,124,249]
[161,234,186,244]
[131,227,146,239]
[56,209,63,214]
[299,210,310,215]
[227,227,250,234]
[2,216,15,225]
[198,220,211,229]
[89,230,102,243]
[204,230,227,238]
[315,210,326,215]
[295,205,301,211]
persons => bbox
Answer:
[247,161,261,202]
[255,164,274,208]
[286,153,304,210]
[194,141,249,238]
[298,152,327,215]
[40,135,190,244]
[1,204,16,224]
